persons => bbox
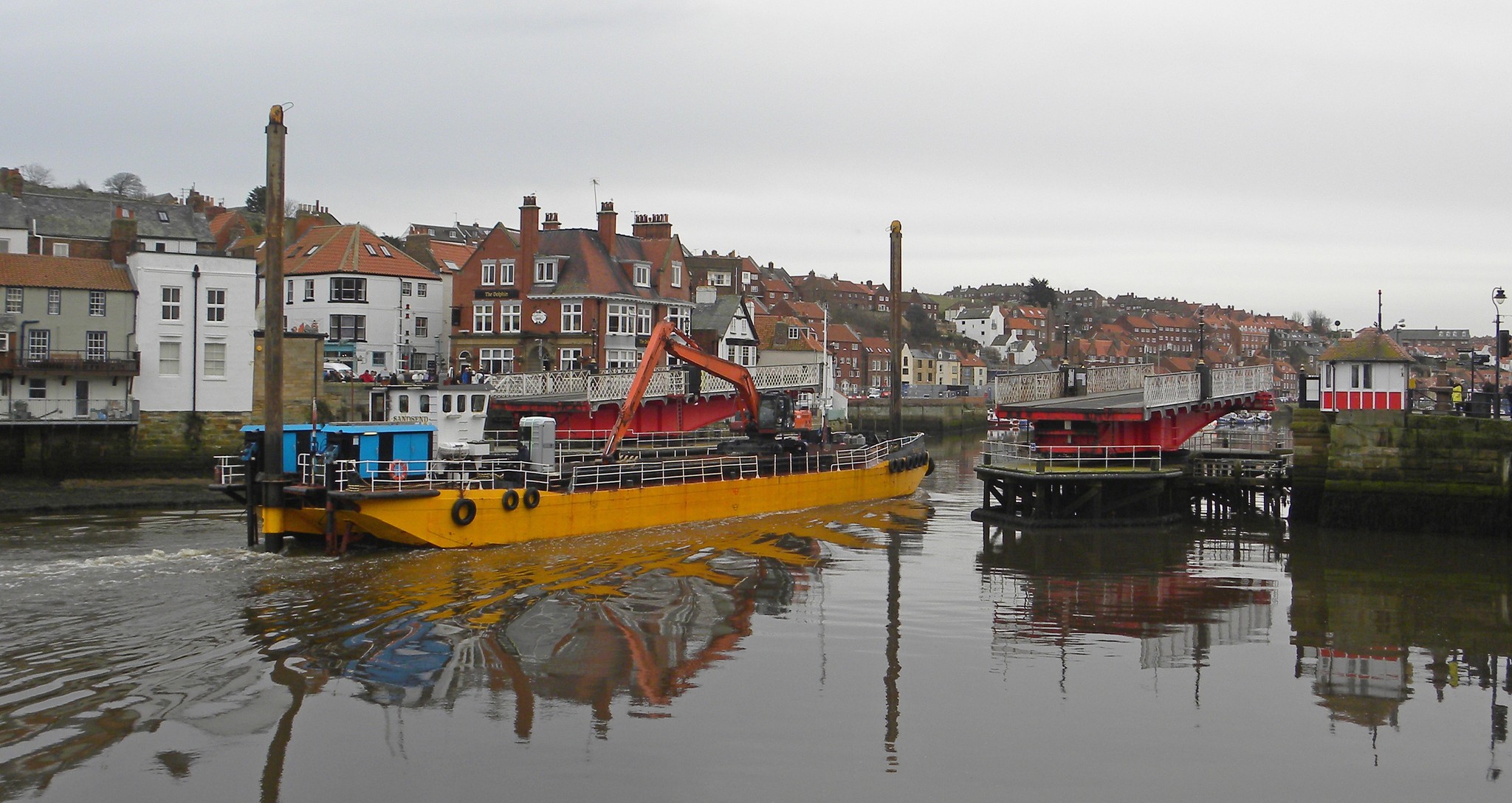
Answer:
[321,357,510,391]
[845,378,893,397]
[1218,410,1272,427]
[1409,370,1512,421]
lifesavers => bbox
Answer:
[388,458,408,481]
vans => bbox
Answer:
[324,361,358,384]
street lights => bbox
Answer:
[1061,323,1069,366]
[1489,286,1506,416]
[1197,309,1207,365]
[1394,318,1405,344]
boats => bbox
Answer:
[212,415,936,550]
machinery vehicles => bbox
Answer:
[597,320,813,460]
